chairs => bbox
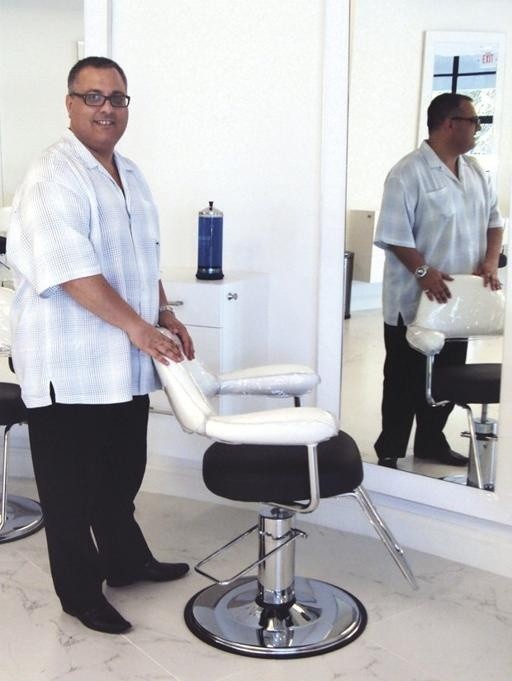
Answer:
[405,272,505,492]
[150,332,418,661]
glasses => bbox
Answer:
[451,117,481,126]
[69,92,130,109]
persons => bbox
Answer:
[5,55,196,634]
[370,91,507,473]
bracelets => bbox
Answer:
[158,304,178,315]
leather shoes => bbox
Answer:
[76,594,132,634]
[418,449,469,467]
[108,558,189,587]
[378,456,398,469]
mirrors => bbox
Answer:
[319,6,508,528]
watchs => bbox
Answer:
[414,265,427,280]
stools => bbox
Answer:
[0,382,45,544]
